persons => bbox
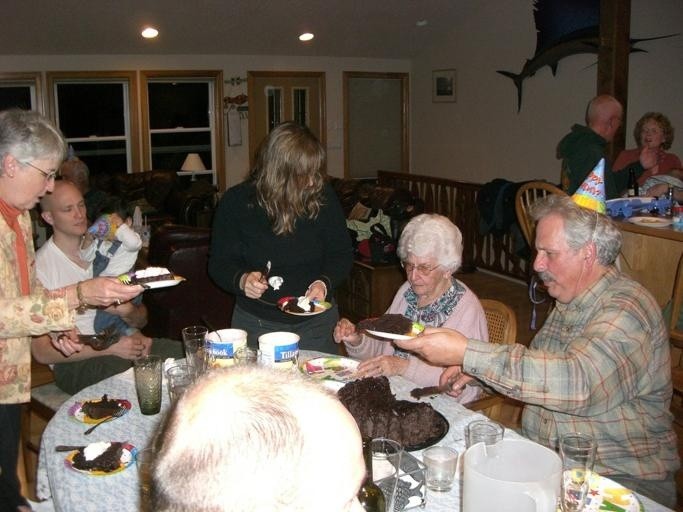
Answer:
[30,178,185,397]
[392,193,682,511]
[148,361,368,512]
[58,157,107,220]
[332,210,491,407]
[611,110,682,205]
[77,195,153,349]
[0,108,147,512]
[555,94,658,204]
[206,118,356,355]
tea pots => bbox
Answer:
[462,438,564,512]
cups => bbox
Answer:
[468,418,505,450]
[168,366,196,400]
[554,432,596,512]
[374,438,458,508]
[183,325,207,370]
[134,354,164,414]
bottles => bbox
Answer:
[669,207,683,232]
[627,165,640,199]
[256,330,300,363]
[207,328,247,367]
[358,433,386,511]
[663,181,675,216]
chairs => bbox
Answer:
[18,319,74,505]
[353,299,535,431]
[517,181,575,304]
[664,249,683,430]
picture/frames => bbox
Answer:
[430,67,457,105]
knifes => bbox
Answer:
[411,381,466,398]
[257,261,272,285]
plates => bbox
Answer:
[556,469,646,512]
[116,272,188,291]
[297,355,369,386]
[66,444,138,476]
[65,397,132,428]
[276,297,333,316]
[628,216,674,230]
[357,318,426,340]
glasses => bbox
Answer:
[15,159,58,181]
[398,260,443,277]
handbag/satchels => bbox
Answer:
[368,223,401,267]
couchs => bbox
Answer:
[82,167,423,335]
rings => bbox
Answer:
[117,297,122,305]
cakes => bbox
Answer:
[337,375,444,449]
[81,399,119,420]
[374,314,412,335]
[72,441,123,471]
[283,295,315,313]
[131,266,175,285]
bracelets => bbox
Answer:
[76,281,96,315]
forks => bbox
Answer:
[84,403,129,435]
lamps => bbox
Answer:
[180,152,204,179]
